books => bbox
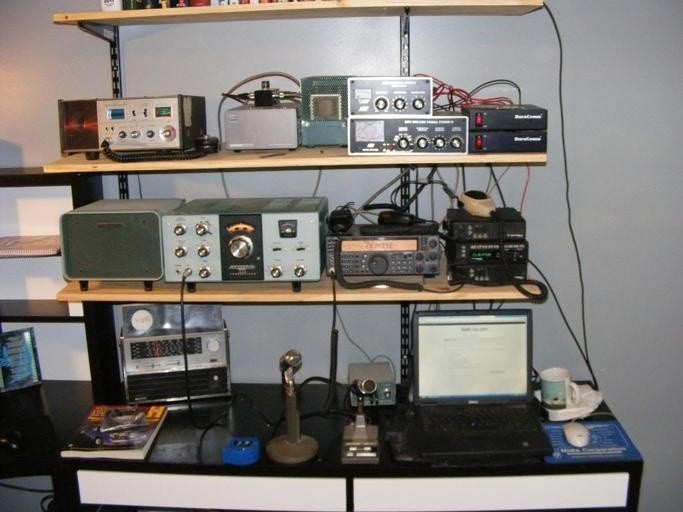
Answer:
[58,397,170,465]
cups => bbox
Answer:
[538,367,580,408]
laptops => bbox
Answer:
[412,309,553,461]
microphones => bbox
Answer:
[351,374,378,398]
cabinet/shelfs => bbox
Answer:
[44,0,549,306]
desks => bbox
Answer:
[55,382,644,512]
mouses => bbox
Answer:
[562,423,591,448]
